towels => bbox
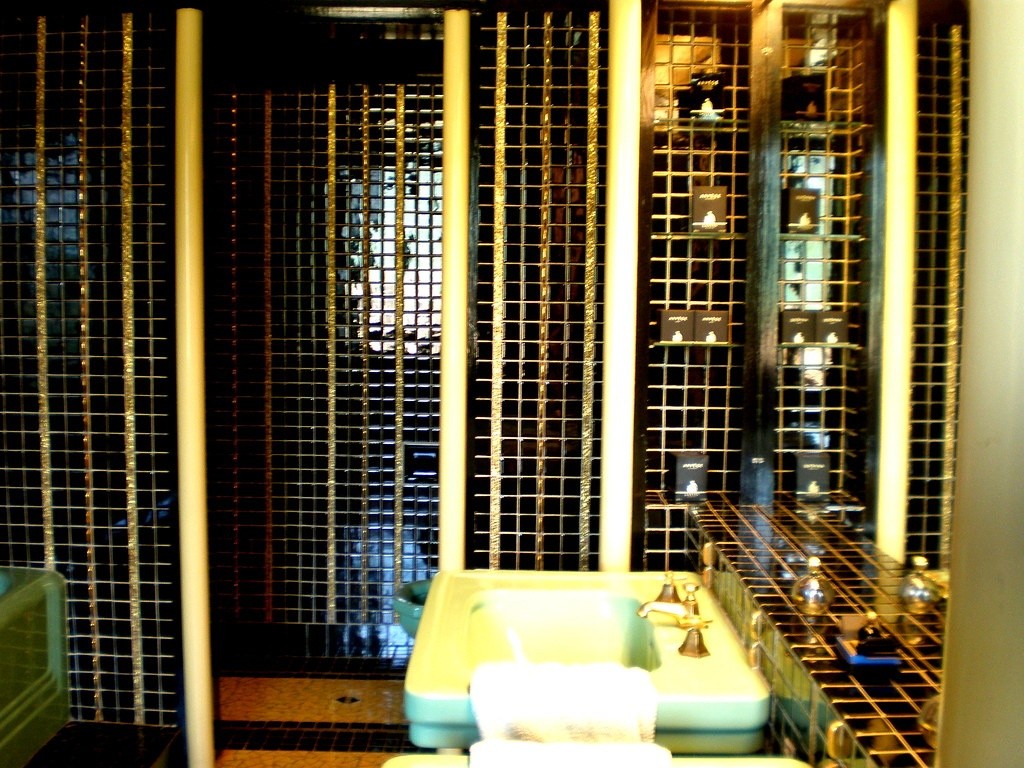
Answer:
[467,662,673,767]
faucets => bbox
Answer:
[636,582,700,630]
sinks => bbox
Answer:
[404,569,771,755]
